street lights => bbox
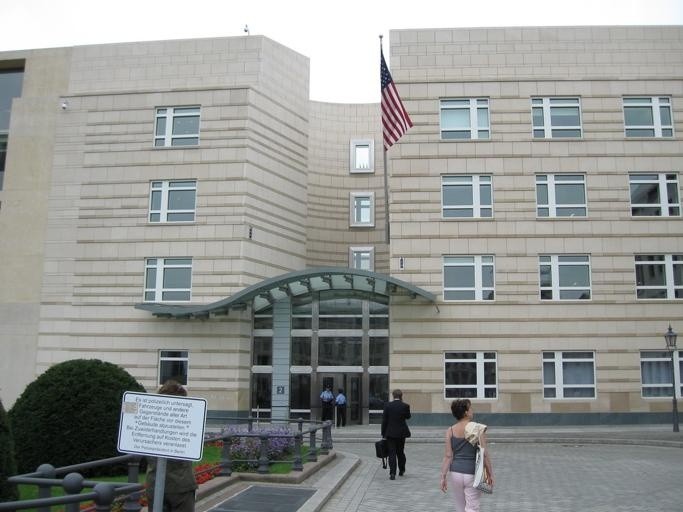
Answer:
[663,319,679,431]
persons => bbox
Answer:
[381,388,414,482]
[144,378,200,512]
[439,398,496,512]
[334,388,347,428]
[320,384,334,425]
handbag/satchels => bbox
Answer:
[373,436,389,458]
[472,443,493,495]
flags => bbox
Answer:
[381,47,415,155]
